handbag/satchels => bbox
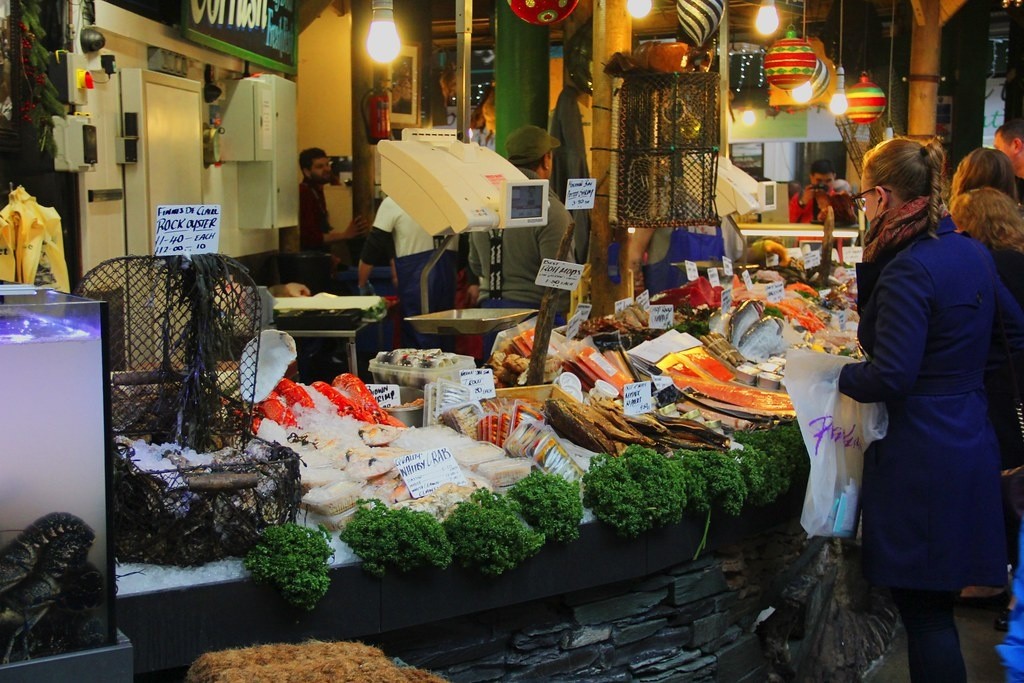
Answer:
[781,347,887,540]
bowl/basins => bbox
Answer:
[553,373,622,406]
[284,424,537,531]
[379,386,424,428]
[735,356,789,394]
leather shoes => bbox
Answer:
[993,606,1012,632]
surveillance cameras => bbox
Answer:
[204,84,222,102]
[80,29,105,53]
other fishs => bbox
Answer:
[542,394,733,455]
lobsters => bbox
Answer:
[236,372,394,433]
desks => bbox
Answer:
[228,321,367,378]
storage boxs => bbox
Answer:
[369,354,476,392]
[0,287,134,683]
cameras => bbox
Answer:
[814,183,829,192]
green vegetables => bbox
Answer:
[670,260,861,359]
[244,419,812,613]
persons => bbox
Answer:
[789,158,858,227]
[245,195,460,382]
[949,124,1024,630]
[839,136,1024,683]
[627,204,792,298]
[298,148,372,251]
[467,125,598,361]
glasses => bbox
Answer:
[852,187,892,213]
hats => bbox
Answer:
[506,124,560,163]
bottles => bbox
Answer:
[659,403,725,436]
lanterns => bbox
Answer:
[506,0,578,25]
[844,72,887,124]
[765,25,839,116]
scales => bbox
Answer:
[379,129,553,335]
[652,153,778,278]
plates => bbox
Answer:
[424,377,587,484]
